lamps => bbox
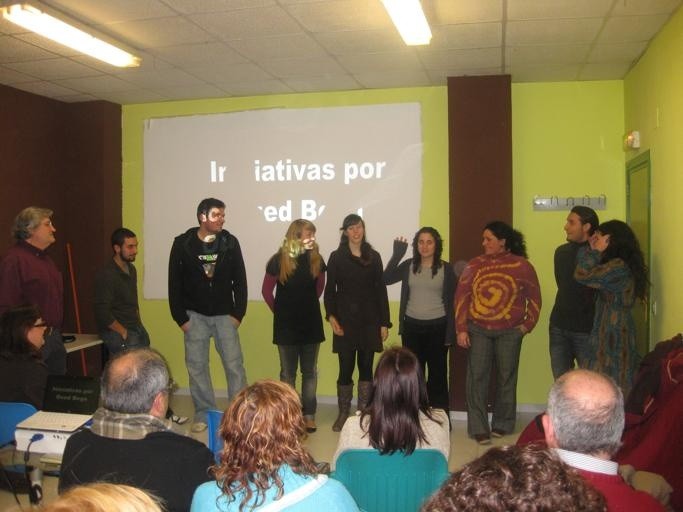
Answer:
[0,0,143,68]
[382,0,433,47]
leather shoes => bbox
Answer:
[306,425,317,433]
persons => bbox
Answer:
[322,213,391,433]
[539,366,669,511]
[330,343,453,471]
[547,205,606,384]
[165,196,248,433]
[0,300,55,412]
[571,217,653,403]
[259,216,326,435]
[56,345,220,512]
[380,225,458,434]
[451,220,542,446]
[0,204,82,375]
[90,226,188,427]
[419,437,612,512]
[188,376,361,512]
[8,481,168,512]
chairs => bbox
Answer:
[205,410,226,465]
[0,402,37,512]
[329,449,453,512]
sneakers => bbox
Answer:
[490,430,503,438]
[471,433,490,444]
[191,422,207,433]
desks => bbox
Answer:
[63,333,104,371]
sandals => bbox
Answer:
[168,414,189,425]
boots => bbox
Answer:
[332,379,354,432]
[357,378,374,413]
